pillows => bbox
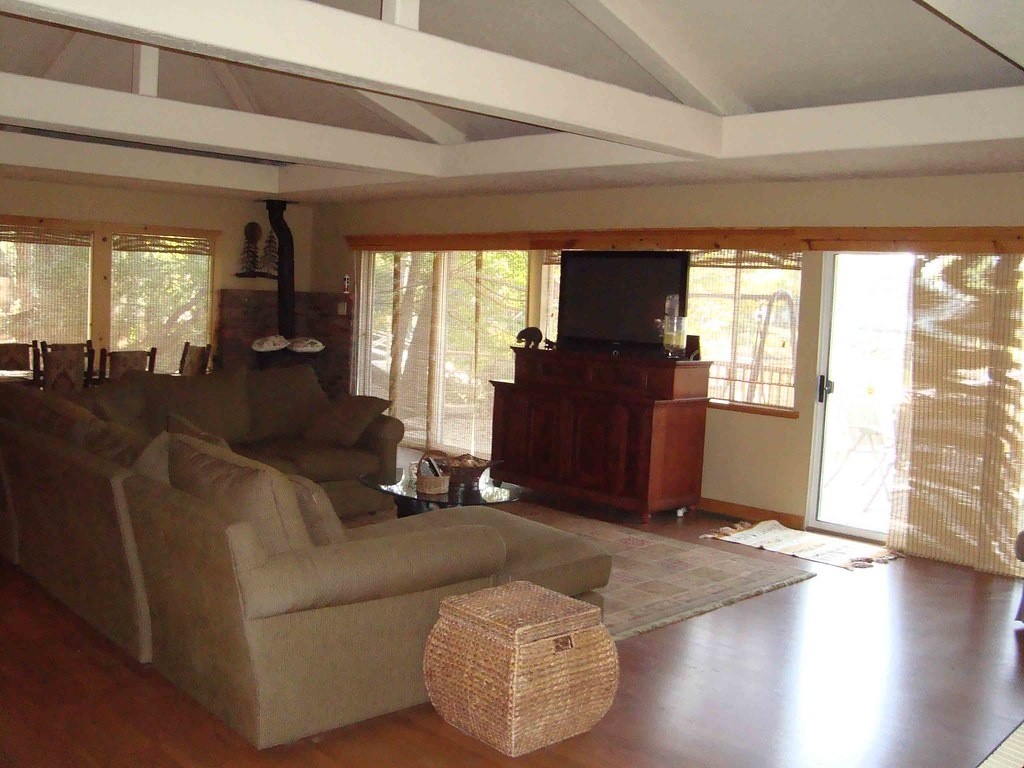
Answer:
[168,431,314,554]
[302,394,392,448]
[285,473,348,547]
[166,369,252,444]
[244,365,329,441]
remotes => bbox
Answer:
[421,457,442,477]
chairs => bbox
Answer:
[99,347,157,385]
[33,349,95,392]
[179,342,212,375]
[0,340,38,372]
[40,340,93,372]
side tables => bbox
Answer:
[423,580,620,757]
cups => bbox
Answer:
[664,316,687,349]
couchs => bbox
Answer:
[0,364,611,750]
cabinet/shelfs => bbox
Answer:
[489,346,714,523]
[214,289,355,396]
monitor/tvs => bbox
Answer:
[558,251,690,352]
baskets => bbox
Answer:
[416,450,450,494]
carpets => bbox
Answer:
[698,519,906,571]
[340,500,817,641]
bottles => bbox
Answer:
[344,275,350,293]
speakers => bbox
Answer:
[686,335,701,360]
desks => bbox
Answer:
[358,466,523,520]
